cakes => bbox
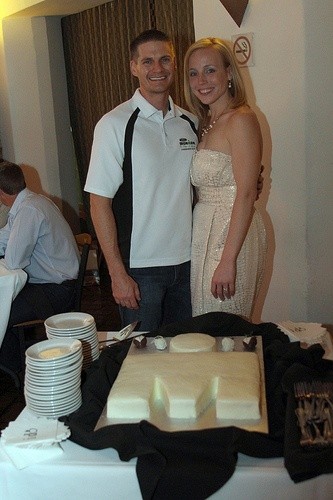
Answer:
[169,333,217,352]
[105,351,261,420]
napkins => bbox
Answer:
[276,318,327,344]
[0,414,71,470]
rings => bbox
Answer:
[223,288,228,291]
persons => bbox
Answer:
[182,37,266,337]
[82,30,202,336]
[0,160,81,390]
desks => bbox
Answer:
[0,321,333,500]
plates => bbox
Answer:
[44,312,101,370]
[24,338,83,418]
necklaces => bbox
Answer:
[200,107,223,136]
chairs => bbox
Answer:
[13,238,91,380]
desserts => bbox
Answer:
[133,335,147,347]
[241,335,257,349]
[153,335,167,350]
[222,337,234,351]
